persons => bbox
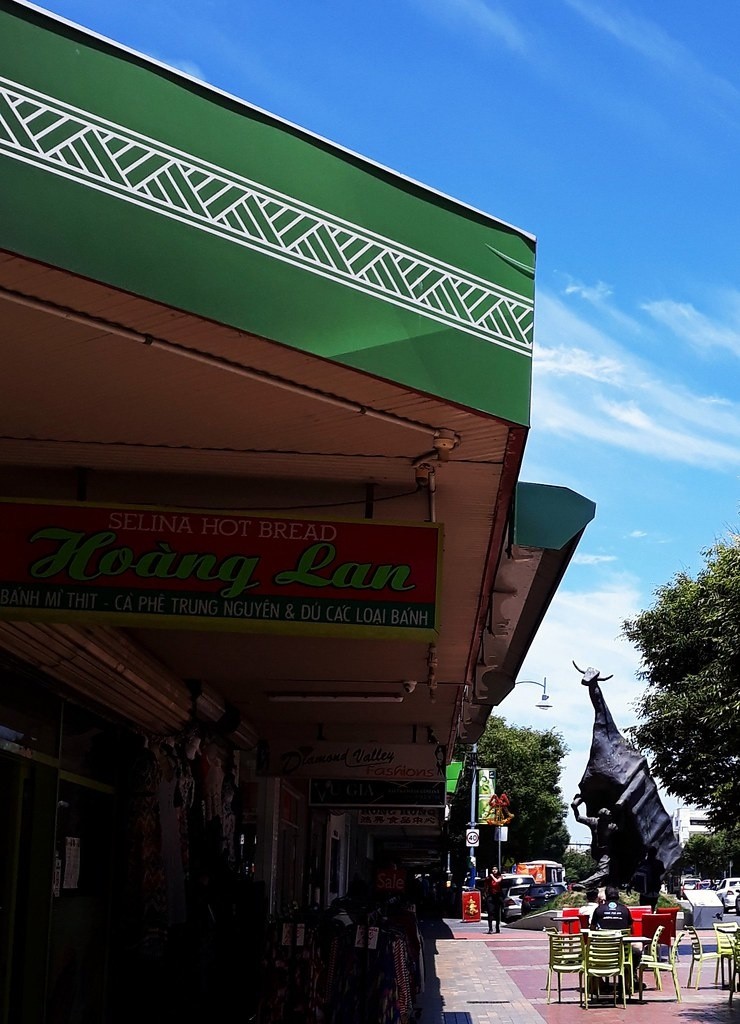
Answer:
[577,887,647,994]
[679,877,720,891]
[626,847,666,915]
[571,803,622,888]
[420,866,505,935]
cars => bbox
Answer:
[660,874,740,916]
[502,859,567,922]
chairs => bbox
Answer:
[685,925,732,990]
[589,931,624,1007]
[584,937,626,1010]
[543,927,582,990]
[594,928,636,998]
[548,933,587,1006]
[718,927,740,1005]
[639,932,686,1003]
[640,926,665,991]
[713,922,740,985]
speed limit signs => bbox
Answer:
[466,829,479,847]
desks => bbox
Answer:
[580,936,653,1002]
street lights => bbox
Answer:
[466,676,553,889]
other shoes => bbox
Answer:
[632,982,646,990]
[488,929,493,934]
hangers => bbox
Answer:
[360,892,402,931]
